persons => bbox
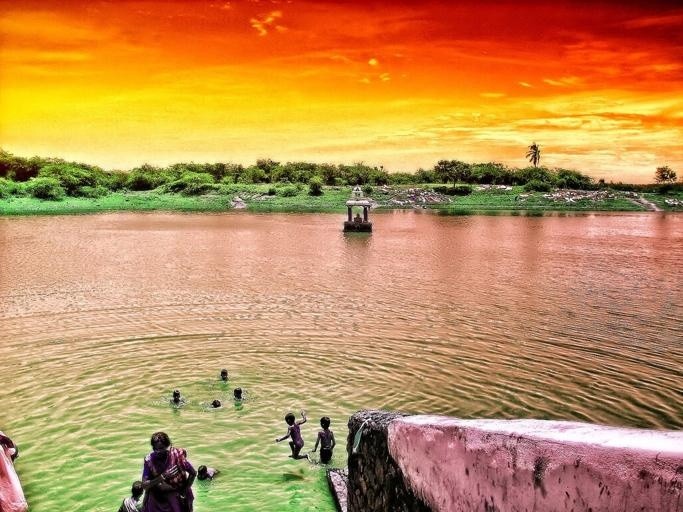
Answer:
[118,432,220,512]
[170,390,184,405]
[211,368,243,408]
[275,411,336,463]
